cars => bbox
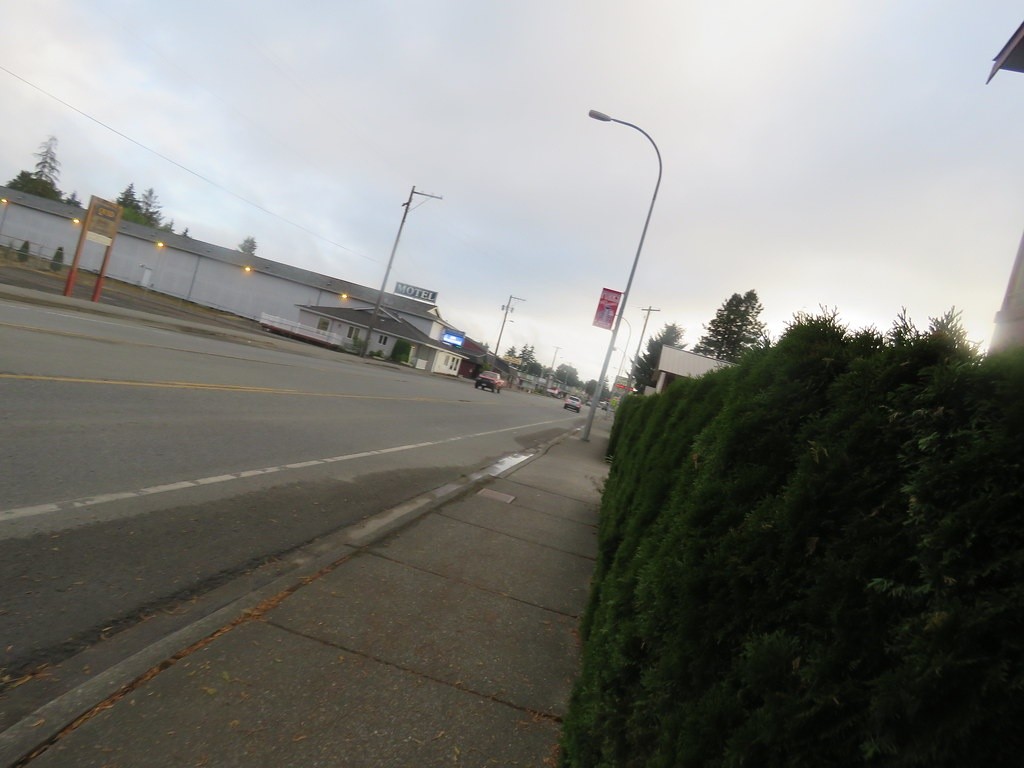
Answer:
[585,401,591,406]
[564,397,581,413]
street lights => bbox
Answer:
[580,109,662,443]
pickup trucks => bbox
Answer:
[475,371,504,393]
